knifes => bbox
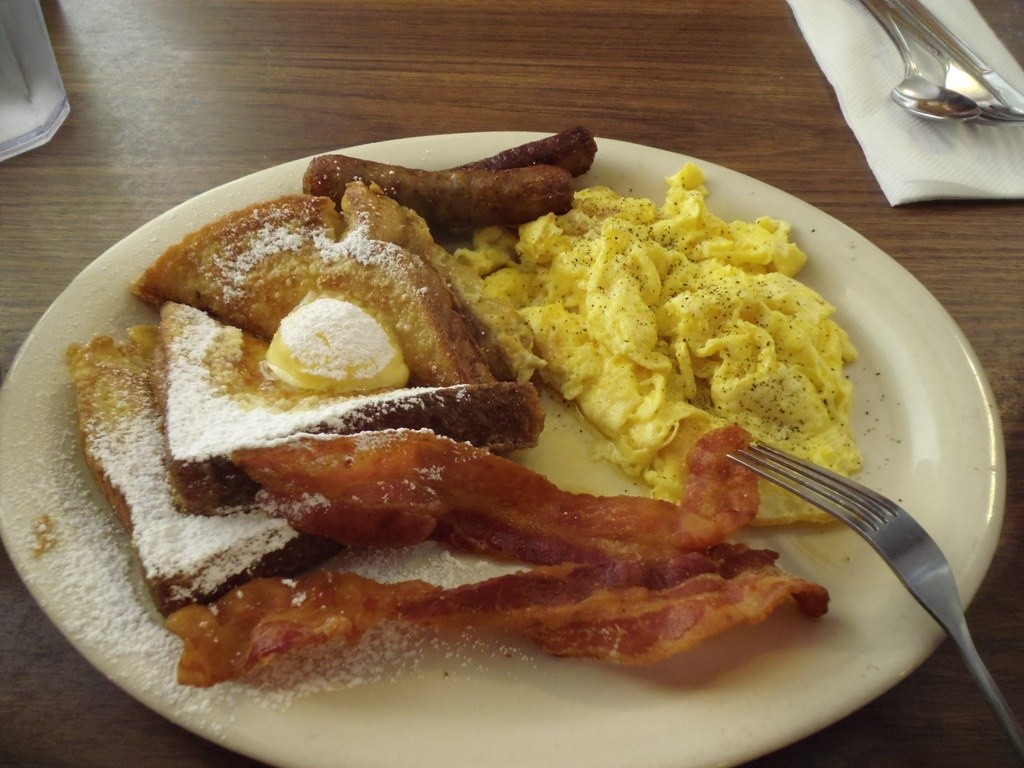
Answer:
[894,0,1024,114]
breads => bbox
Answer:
[62,180,546,615]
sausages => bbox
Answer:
[305,153,578,231]
[449,126,598,178]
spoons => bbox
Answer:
[856,0,981,121]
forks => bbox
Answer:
[727,439,1023,761]
[882,0,1024,123]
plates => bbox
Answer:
[1,130,1007,768]
[1,0,71,161]
